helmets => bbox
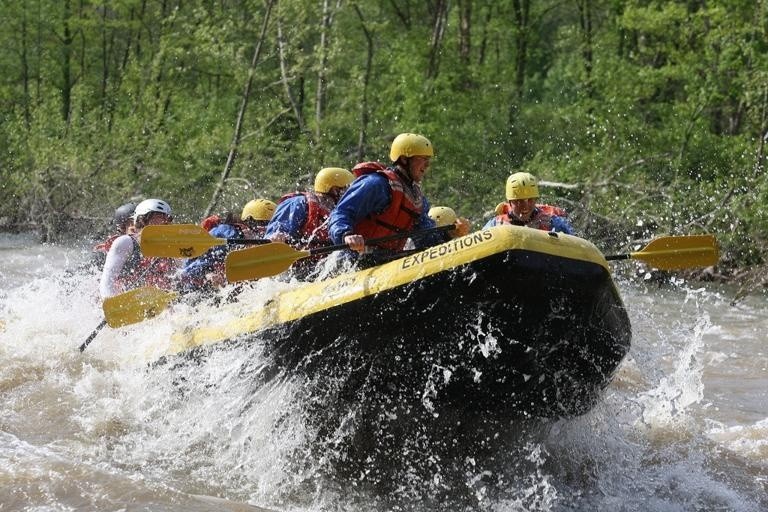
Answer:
[388,132,434,163]
[426,206,457,227]
[312,166,355,194]
[132,198,173,224]
[505,171,540,201]
[112,202,136,220]
[240,195,278,224]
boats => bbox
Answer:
[109,222,633,429]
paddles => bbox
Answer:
[104,284,211,328]
[605,234,720,268]
[141,225,272,260]
[226,222,461,283]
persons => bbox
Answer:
[480,171,574,236]
[64,134,470,324]
